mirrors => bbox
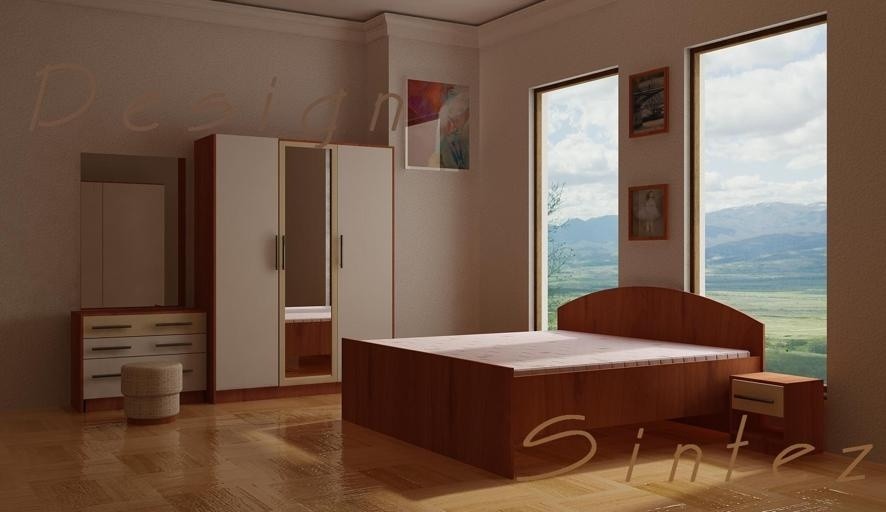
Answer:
[279,140,337,387]
[80,152,179,310]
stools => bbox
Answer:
[121,360,184,425]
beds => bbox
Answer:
[340,286,765,479]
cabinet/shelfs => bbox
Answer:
[194,132,395,405]
[71,310,208,414]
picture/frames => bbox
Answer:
[629,183,669,240]
[629,67,668,137]
[404,77,471,172]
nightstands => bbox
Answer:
[729,372,824,455]
